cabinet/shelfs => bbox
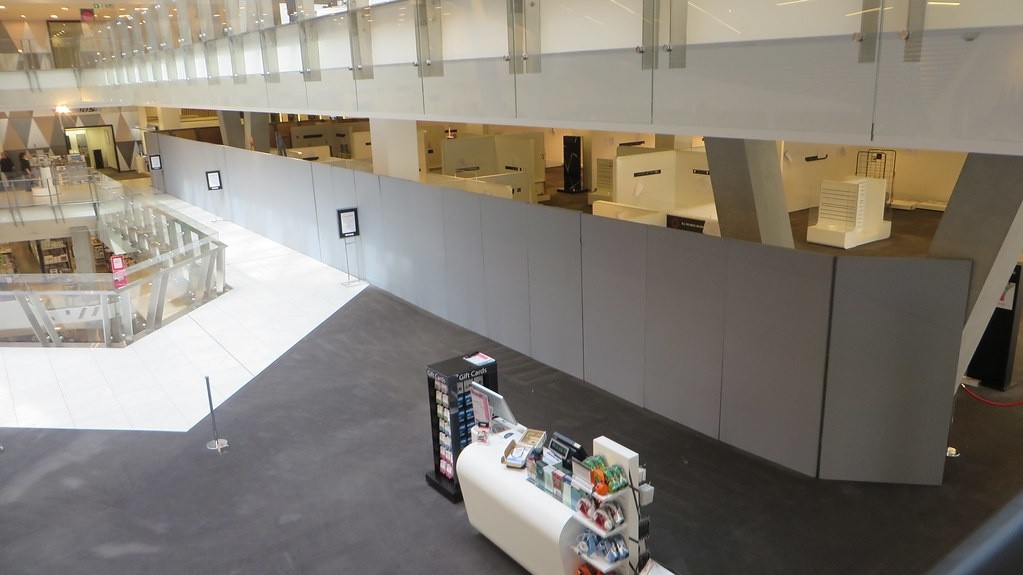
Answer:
[569,434,639,573]
[58,172,69,184]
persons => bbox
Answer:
[18,152,33,192]
